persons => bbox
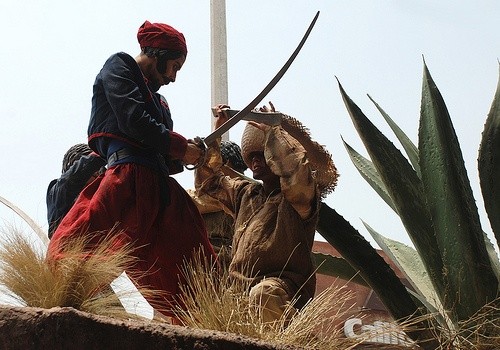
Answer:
[46,20,224,326]
[183,139,249,277]
[196,102,336,329]
[46,143,125,311]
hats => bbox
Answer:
[241,111,340,201]
[218,140,249,174]
[137,19,188,55]
[62,143,93,173]
